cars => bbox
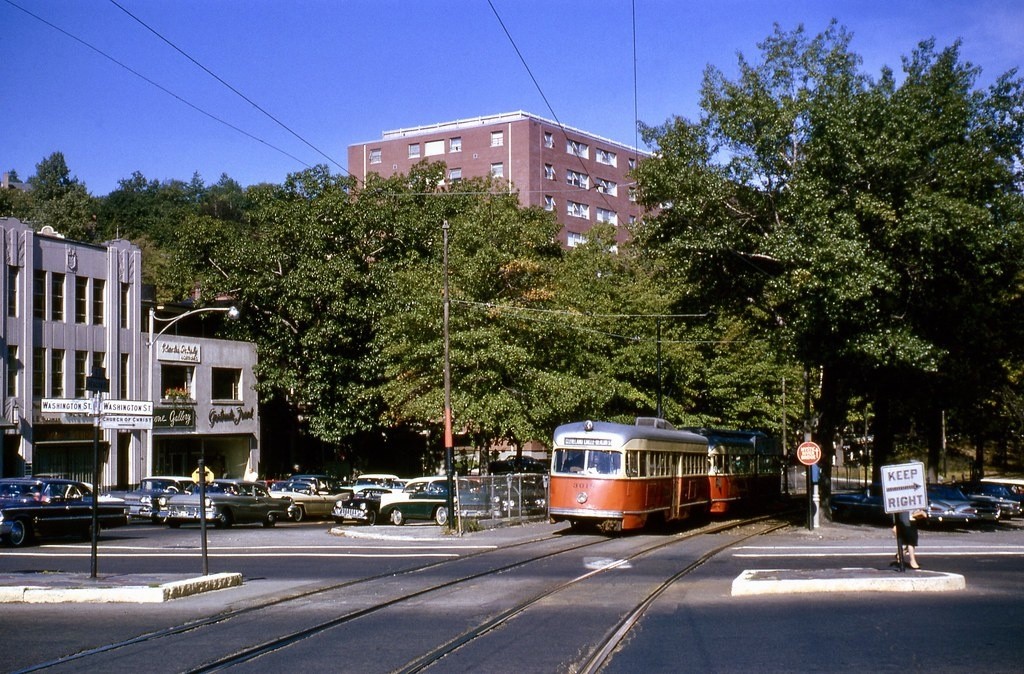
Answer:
[256,456,548,529]
[124,476,200,525]
[0,478,131,548]
[832,479,1024,528]
[159,479,296,529]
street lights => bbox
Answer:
[144,306,239,488]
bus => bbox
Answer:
[677,426,783,515]
[549,420,714,536]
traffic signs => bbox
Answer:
[880,461,929,513]
[99,416,154,430]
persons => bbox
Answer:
[892,508,918,570]
[589,454,604,471]
